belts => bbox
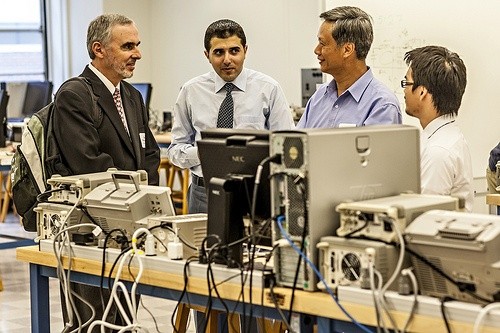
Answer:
[191,172,204,187]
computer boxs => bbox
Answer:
[269,120,426,299]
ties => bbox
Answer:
[113,88,132,144]
[217,83,235,128]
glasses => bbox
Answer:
[401,80,414,89]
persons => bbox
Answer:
[296,6,403,333]
[44,14,160,333]
[165,19,296,333]
[400,46,476,209]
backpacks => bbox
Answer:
[11,77,103,232]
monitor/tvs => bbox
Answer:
[197,124,271,267]
[128,81,152,110]
[21,80,53,117]
